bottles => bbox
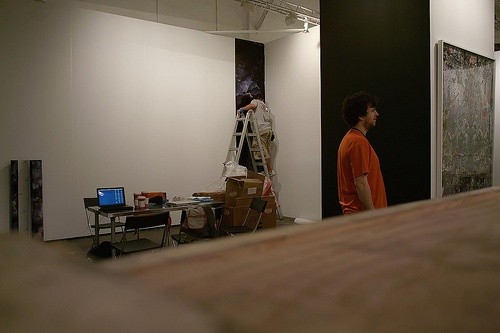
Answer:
[133,192,142,209]
[137,196,145,210]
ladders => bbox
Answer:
[217,108,285,221]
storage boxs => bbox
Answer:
[215,171,277,231]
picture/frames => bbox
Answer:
[437,40,496,197]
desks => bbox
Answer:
[90,200,224,260]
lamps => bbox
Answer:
[285,16,297,25]
[241,2,254,12]
[303,24,309,33]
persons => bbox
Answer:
[337,92,387,216]
[237,84,274,177]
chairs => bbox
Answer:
[83,192,268,258]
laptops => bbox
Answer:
[97,187,134,212]
